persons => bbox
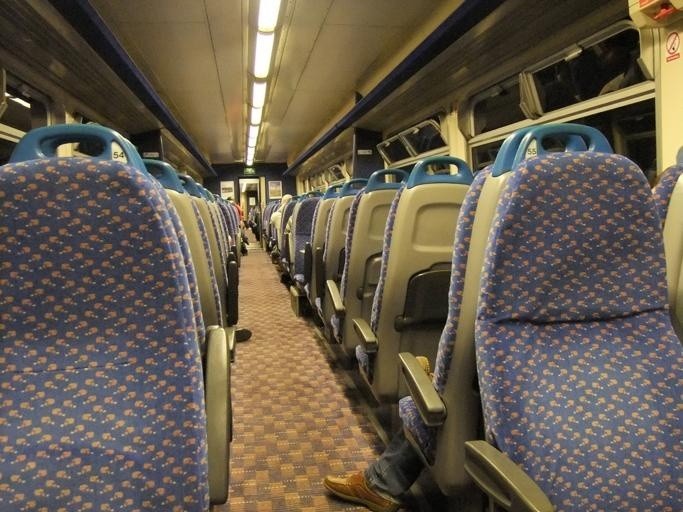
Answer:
[322,418,426,511]
[226,194,293,258]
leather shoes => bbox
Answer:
[323,469,401,511]
